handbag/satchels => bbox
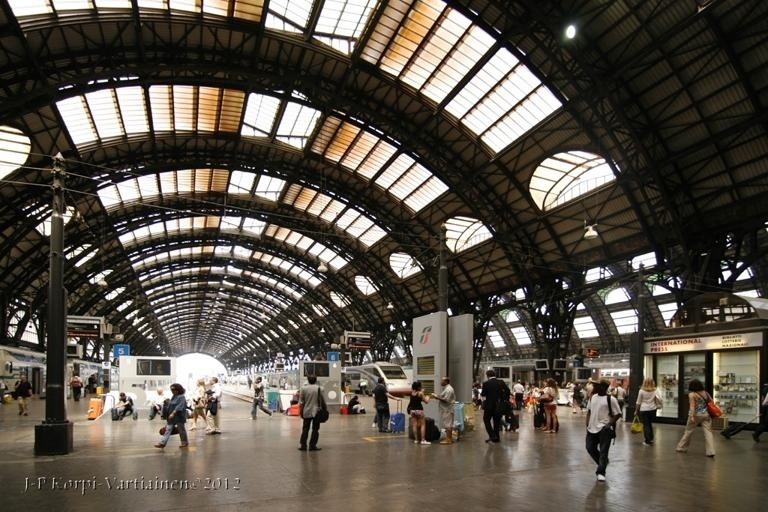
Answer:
[313,406,331,423]
[407,402,411,414]
[610,413,617,440]
[159,426,180,436]
[630,415,644,433]
[707,401,721,419]
[538,392,555,402]
[655,394,663,409]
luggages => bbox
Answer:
[720,413,760,438]
[390,400,406,434]
[409,416,435,441]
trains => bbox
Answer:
[0,344,118,402]
[214,361,413,398]
[584,351,630,383]
[397,357,551,393]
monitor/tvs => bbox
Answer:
[553,359,567,371]
[534,359,549,370]
[573,366,592,383]
[489,365,512,382]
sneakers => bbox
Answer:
[420,439,431,446]
[440,439,452,444]
[178,443,190,449]
[297,444,308,451]
[413,440,419,445]
[642,439,654,446]
[154,443,166,449]
[596,472,605,482]
[308,444,322,451]
[189,424,222,434]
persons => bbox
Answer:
[431,375,456,444]
[633,376,663,447]
[751,391,768,443]
[14,375,32,416]
[112,374,290,449]
[69,376,83,401]
[409,381,432,445]
[342,377,392,433]
[473,369,626,443]
[297,374,327,450]
[675,378,716,457]
[89,375,95,393]
[585,378,623,481]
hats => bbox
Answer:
[594,379,611,386]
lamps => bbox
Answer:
[583,133,598,238]
[317,126,328,274]
[225,285,394,367]
[98,203,107,286]
